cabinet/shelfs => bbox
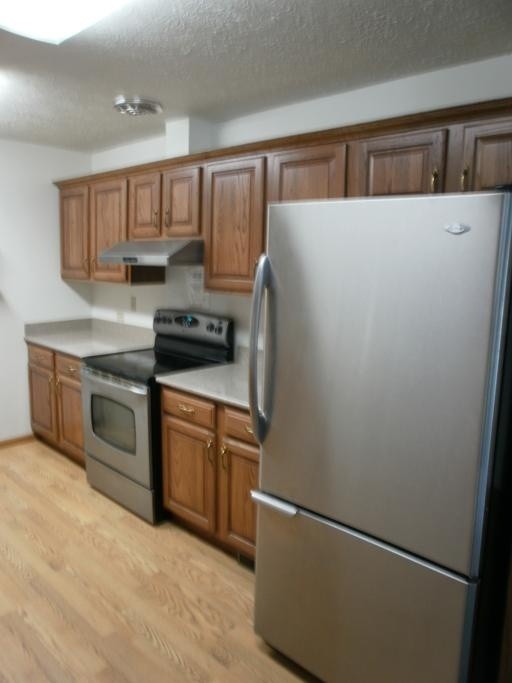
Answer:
[56,169,167,285]
[127,154,201,238]
[202,130,349,294]
[26,331,155,469]
[350,102,512,198]
[156,358,257,566]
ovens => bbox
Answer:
[81,358,166,523]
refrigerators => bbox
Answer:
[248,192,511,682]
